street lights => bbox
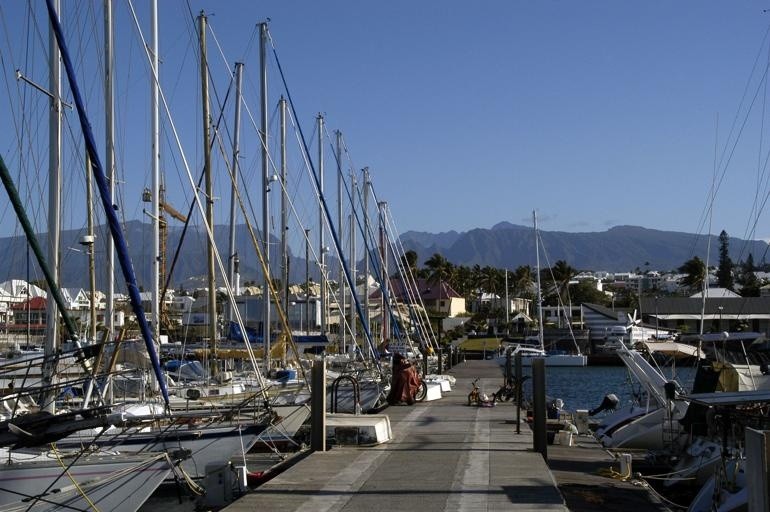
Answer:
[612,296,616,311]
[718,305,723,333]
[655,295,661,340]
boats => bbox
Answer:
[594,331,770,512]
[596,326,682,366]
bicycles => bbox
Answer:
[468,374,516,406]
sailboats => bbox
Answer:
[494,210,588,366]
